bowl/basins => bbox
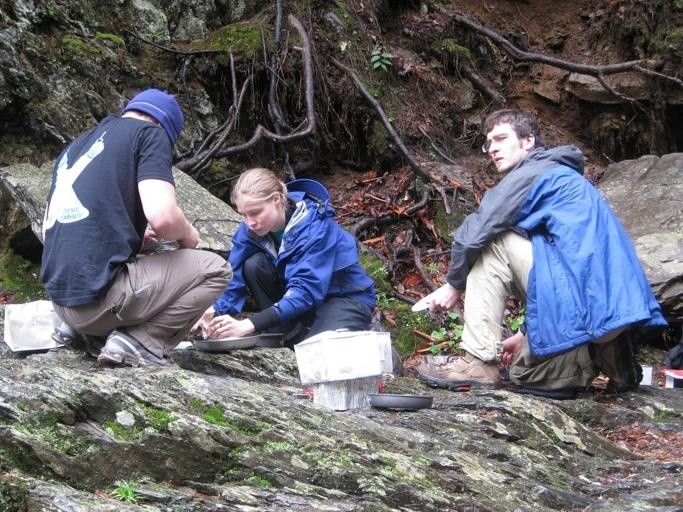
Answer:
[257,333,283,347]
[141,242,178,251]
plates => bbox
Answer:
[368,393,433,408]
[192,336,256,351]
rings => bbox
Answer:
[220,323,222,328]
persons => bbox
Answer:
[199,167,377,340]
[415,107,670,394]
[40,88,234,368]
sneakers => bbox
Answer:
[597,333,644,392]
[414,353,503,392]
[50,319,105,359]
[96,324,181,370]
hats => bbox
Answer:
[119,87,187,151]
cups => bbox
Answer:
[638,364,653,385]
